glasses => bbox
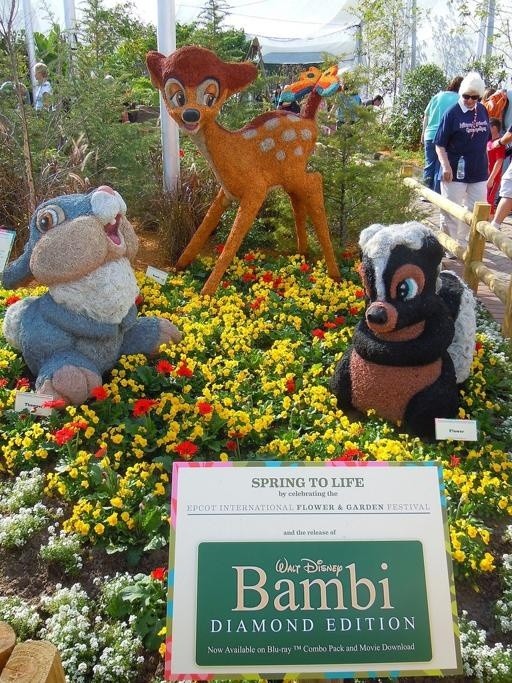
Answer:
[461,94,480,100]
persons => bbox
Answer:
[34,62,53,112]
[420,70,512,259]
[256,82,384,129]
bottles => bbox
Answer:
[456,156,465,179]
